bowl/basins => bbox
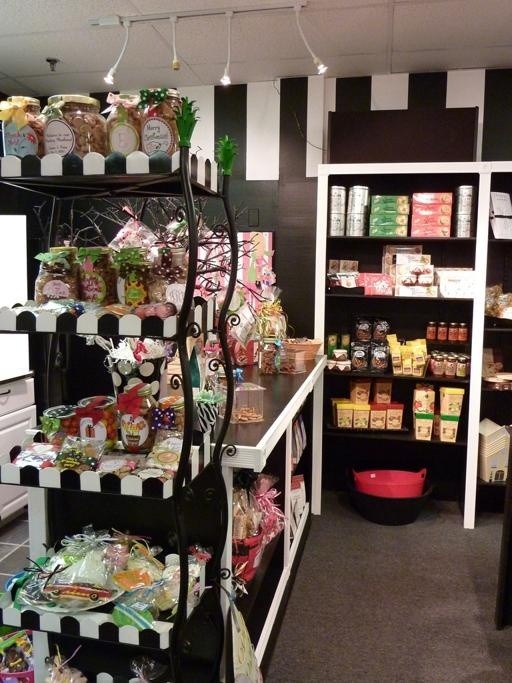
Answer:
[352,486,437,526]
[353,468,428,499]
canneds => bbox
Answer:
[44,405,81,448]
[42,94,108,158]
[437,322,448,341]
[1,95,44,158]
[35,247,188,307]
[136,89,180,156]
[426,321,436,340]
[119,383,155,453]
[457,186,473,238]
[156,397,185,443]
[330,186,346,236]
[81,395,119,451]
[459,323,468,341]
[449,323,458,341]
[105,93,142,155]
[347,186,370,236]
[257,338,282,375]
[431,350,471,380]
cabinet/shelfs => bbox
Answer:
[0,139,238,683]
[0,377,37,524]
[159,355,327,681]
[312,161,512,529]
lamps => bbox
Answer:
[313,58,329,76]
[105,68,115,86]
[221,68,231,87]
